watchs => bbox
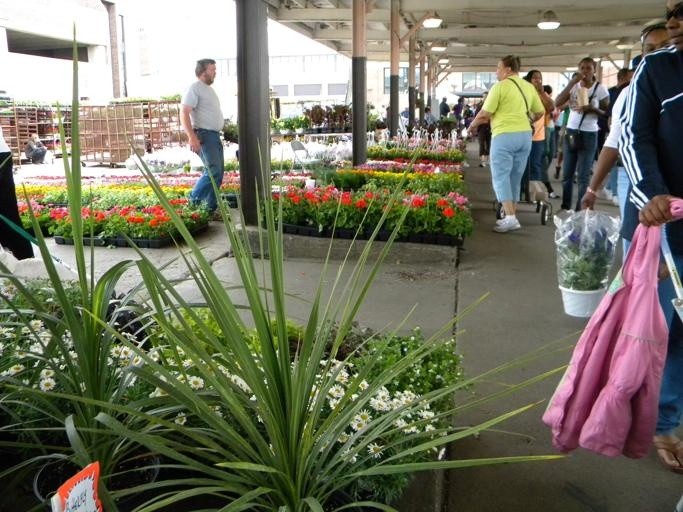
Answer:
[586,186,596,195]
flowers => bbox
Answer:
[556,213,614,291]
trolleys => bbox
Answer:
[495,150,551,226]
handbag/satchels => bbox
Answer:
[564,132,586,153]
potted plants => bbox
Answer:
[0,95,584,512]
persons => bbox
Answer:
[0,126,34,261]
[523,56,640,207]
[400,97,478,142]
[525,70,561,199]
[24,133,46,164]
[475,92,491,167]
[555,56,610,217]
[466,56,546,230]
[179,58,230,222]
[578,21,672,261]
[617,0,682,475]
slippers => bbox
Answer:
[652,438,683,471]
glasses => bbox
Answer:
[643,21,666,34]
[666,5,683,21]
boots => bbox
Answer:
[555,166,560,178]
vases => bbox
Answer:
[559,284,610,316]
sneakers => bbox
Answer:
[604,186,619,207]
[493,218,522,232]
[480,160,489,167]
[189,203,231,221]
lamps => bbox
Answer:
[422,10,442,28]
[537,10,560,30]
